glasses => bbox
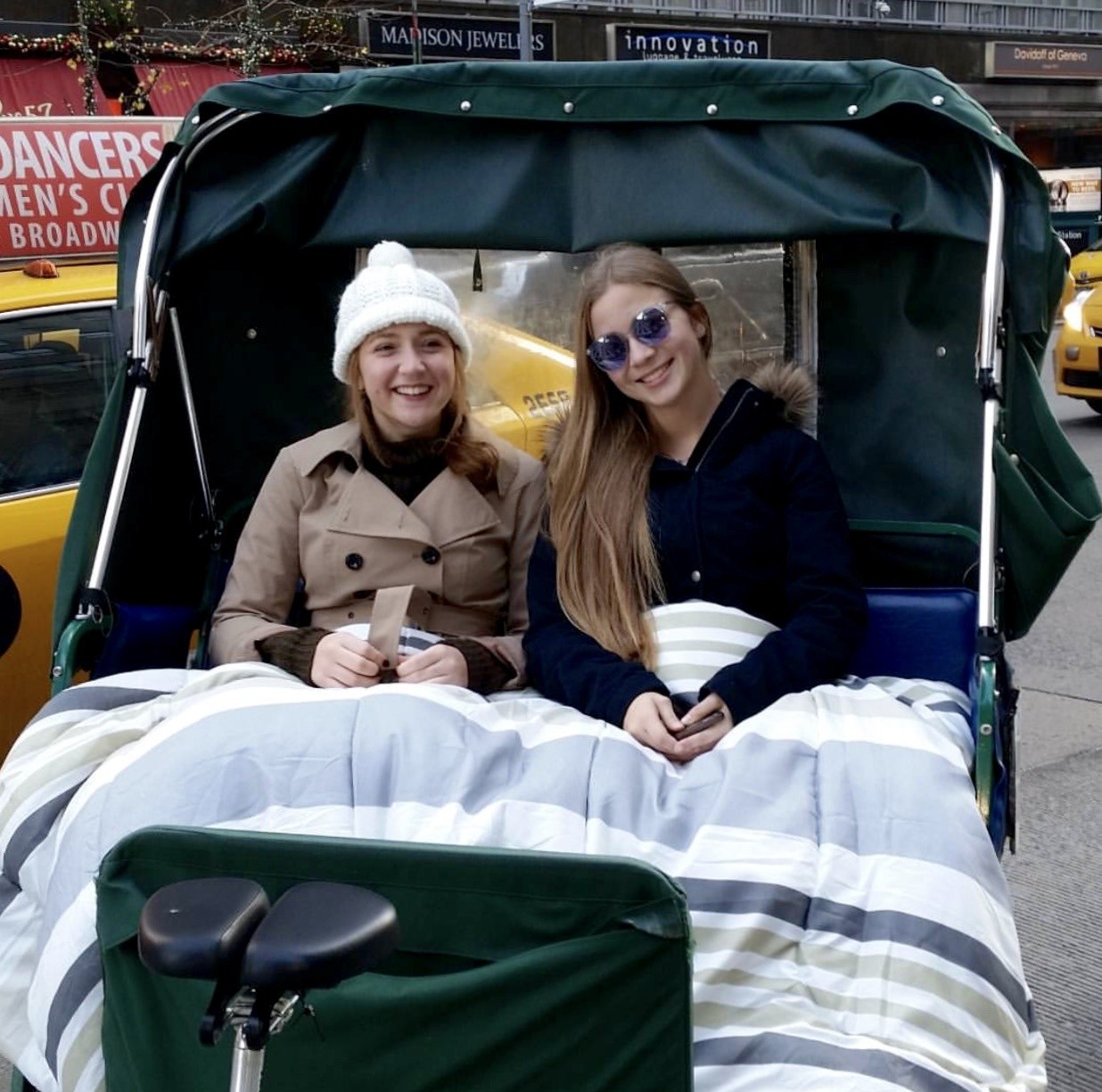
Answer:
[586,299,677,371]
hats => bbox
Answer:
[332,240,472,382]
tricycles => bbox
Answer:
[0,55,1018,1092]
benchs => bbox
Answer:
[101,519,979,828]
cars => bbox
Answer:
[1068,237,1102,290]
[1051,284,1102,415]
[0,113,588,766]
[460,243,786,397]
[1051,270,1076,320]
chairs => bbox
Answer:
[26,341,102,477]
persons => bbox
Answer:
[522,240,868,759]
[206,240,549,694]
